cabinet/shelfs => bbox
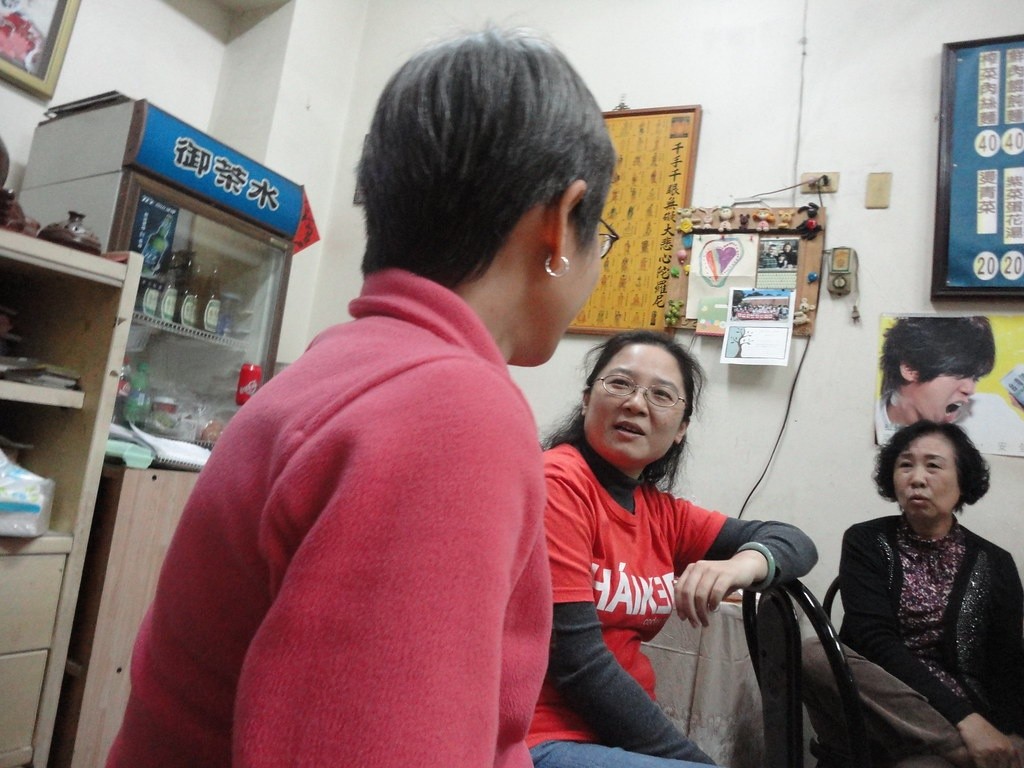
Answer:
[0,224,145,766]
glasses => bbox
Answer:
[596,215,620,258]
[590,373,689,409]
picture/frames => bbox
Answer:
[0,0,82,102]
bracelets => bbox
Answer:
[738,541,776,591]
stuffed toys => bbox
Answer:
[677,202,824,242]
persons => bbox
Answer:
[105,31,620,768]
[874,316,1024,457]
[800,419,1024,768]
[735,305,789,321]
[526,330,819,768]
[761,243,797,268]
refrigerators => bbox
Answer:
[15,92,305,485]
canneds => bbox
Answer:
[149,396,176,417]
[236,363,262,405]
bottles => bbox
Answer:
[130,214,175,275]
[102,247,229,434]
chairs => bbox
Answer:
[740,573,871,768]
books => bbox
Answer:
[110,419,212,472]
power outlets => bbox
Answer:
[799,170,840,193]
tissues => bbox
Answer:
[0,447,57,538]
